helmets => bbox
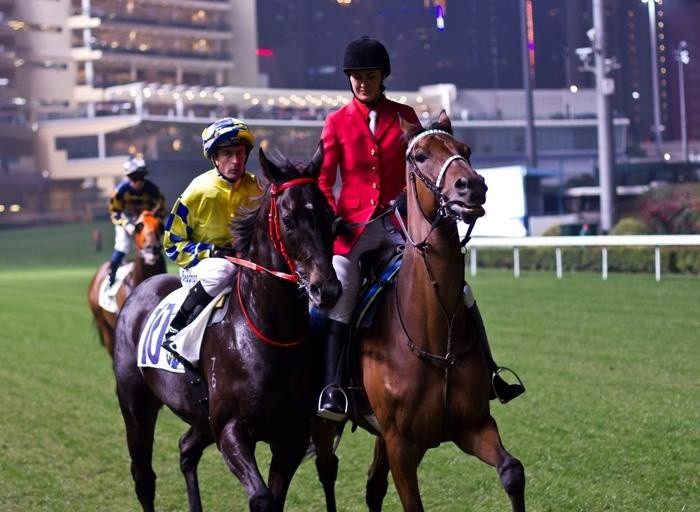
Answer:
[342,36,390,71]
[124,158,146,176]
[202,117,256,160]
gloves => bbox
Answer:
[334,216,351,240]
[211,241,236,259]
[392,191,407,216]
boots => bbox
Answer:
[163,281,215,354]
[467,299,526,401]
[318,316,353,413]
[106,261,120,287]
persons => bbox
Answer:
[102,156,168,290]
[312,34,524,416]
[159,116,268,371]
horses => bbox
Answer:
[87,196,167,361]
[112,136,345,512]
[300,109,527,512]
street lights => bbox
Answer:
[679,41,690,163]
